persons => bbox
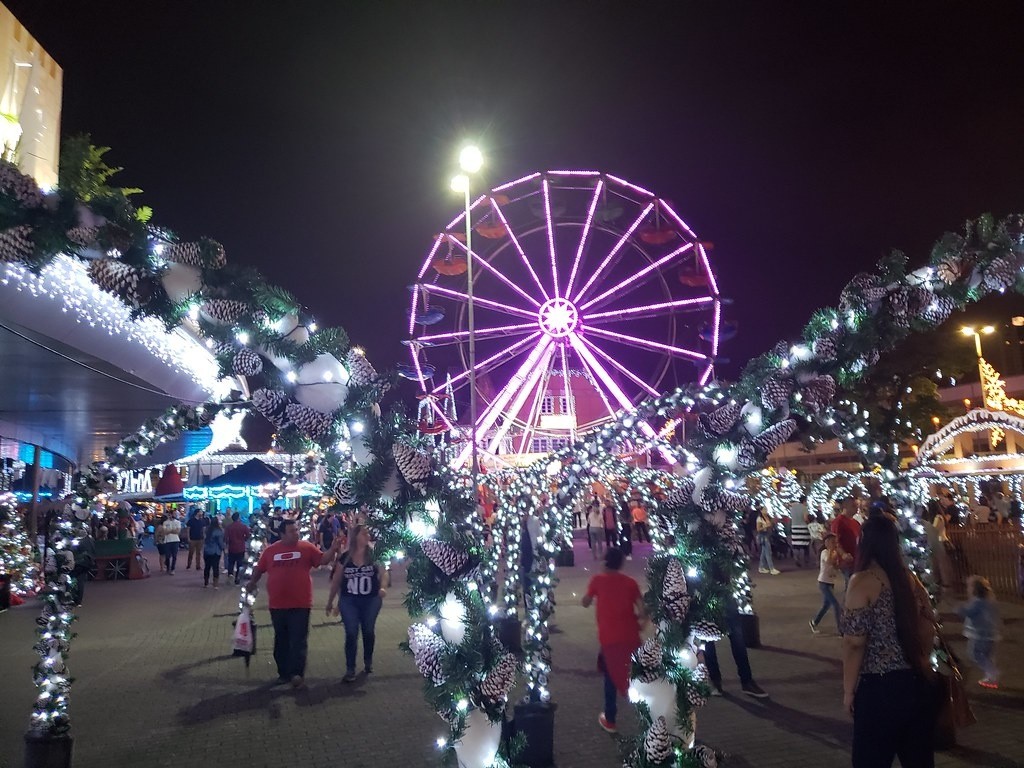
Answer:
[94,507,250,589]
[325,525,389,682]
[919,492,961,588]
[246,520,339,685]
[569,491,652,561]
[702,621,769,699]
[843,512,936,768]
[262,507,362,570]
[948,576,999,687]
[963,492,1024,524]
[741,495,810,575]
[809,495,869,636]
[582,548,646,732]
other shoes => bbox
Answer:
[364,665,373,673]
[770,568,780,575]
[759,568,769,573]
[75,603,81,607]
[743,684,767,698]
[978,677,1000,688]
[712,687,722,696]
[809,620,820,635]
[277,675,289,683]
[343,672,355,682]
[291,675,303,686]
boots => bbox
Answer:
[213,578,219,590]
[204,577,209,587]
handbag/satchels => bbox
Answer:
[922,609,977,730]
[232,606,253,653]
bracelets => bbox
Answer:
[381,588,385,591]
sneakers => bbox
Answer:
[599,712,617,733]
